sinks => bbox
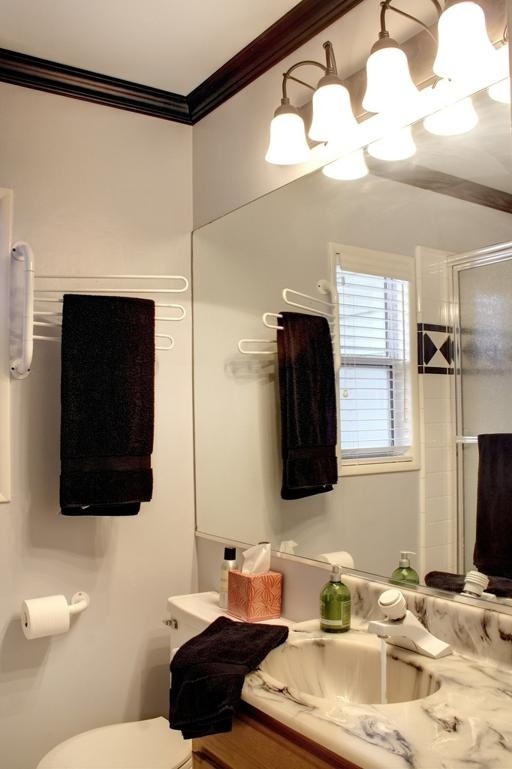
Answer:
[263,636,441,707]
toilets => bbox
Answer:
[40,594,290,769]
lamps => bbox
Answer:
[264,0,500,168]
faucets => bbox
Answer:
[368,590,452,660]
[462,570,493,597]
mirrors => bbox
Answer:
[193,75,512,615]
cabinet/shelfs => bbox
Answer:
[192,700,361,769]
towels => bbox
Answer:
[425,571,512,597]
[170,615,291,738]
[61,294,156,514]
[472,433,512,600]
[277,311,337,500]
